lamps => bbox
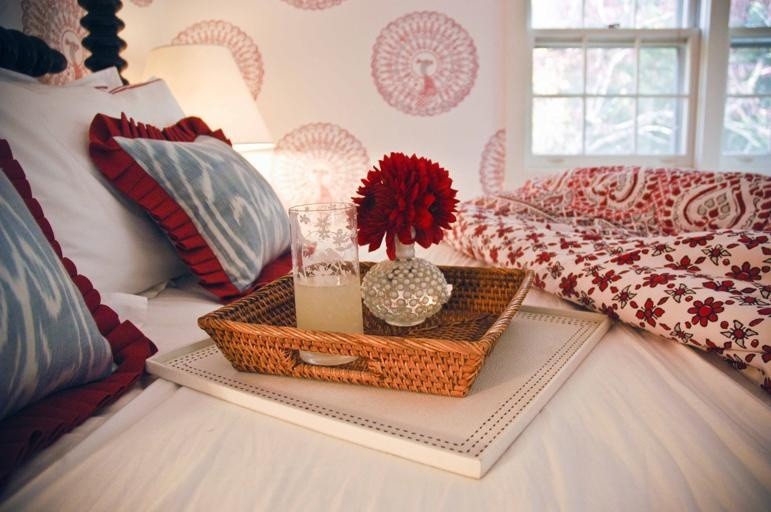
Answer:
[144,42,277,152]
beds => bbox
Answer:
[0,220,771,512]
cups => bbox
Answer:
[288,202,364,366]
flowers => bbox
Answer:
[345,151,463,264]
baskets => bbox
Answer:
[197,259,534,399]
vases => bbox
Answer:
[359,226,456,327]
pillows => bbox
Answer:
[0,138,160,486]
[85,110,309,303]
[0,74,200,302]
